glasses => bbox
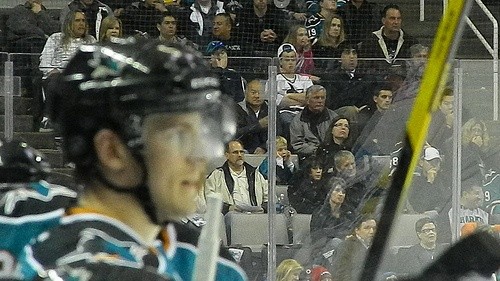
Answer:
[419,228,436,233]
[229,150,246,155]
[334,123,349,128]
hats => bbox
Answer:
[424,147,442,161]
[299,268,312,279]
[206,41,226,54]
[277,43,297,59]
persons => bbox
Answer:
[0,0,500,281]
[14,40,224,281]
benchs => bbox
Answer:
[221,154,437,248]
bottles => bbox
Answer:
[279,193,286,212]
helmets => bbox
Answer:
[63,39,221,149]
[0,139,42,182]
